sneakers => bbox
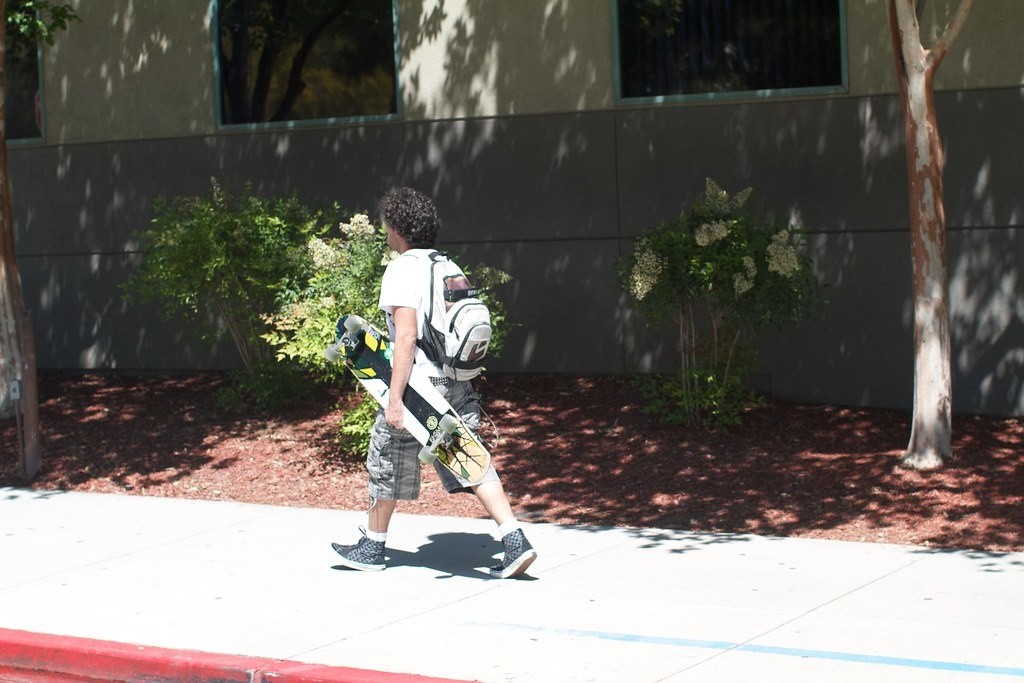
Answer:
[490,524,537,578]
[330,525,387,570]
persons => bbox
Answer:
[331,187,537,579]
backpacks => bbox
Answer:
[402,248,492,382]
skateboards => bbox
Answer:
[322,313,493,485]
